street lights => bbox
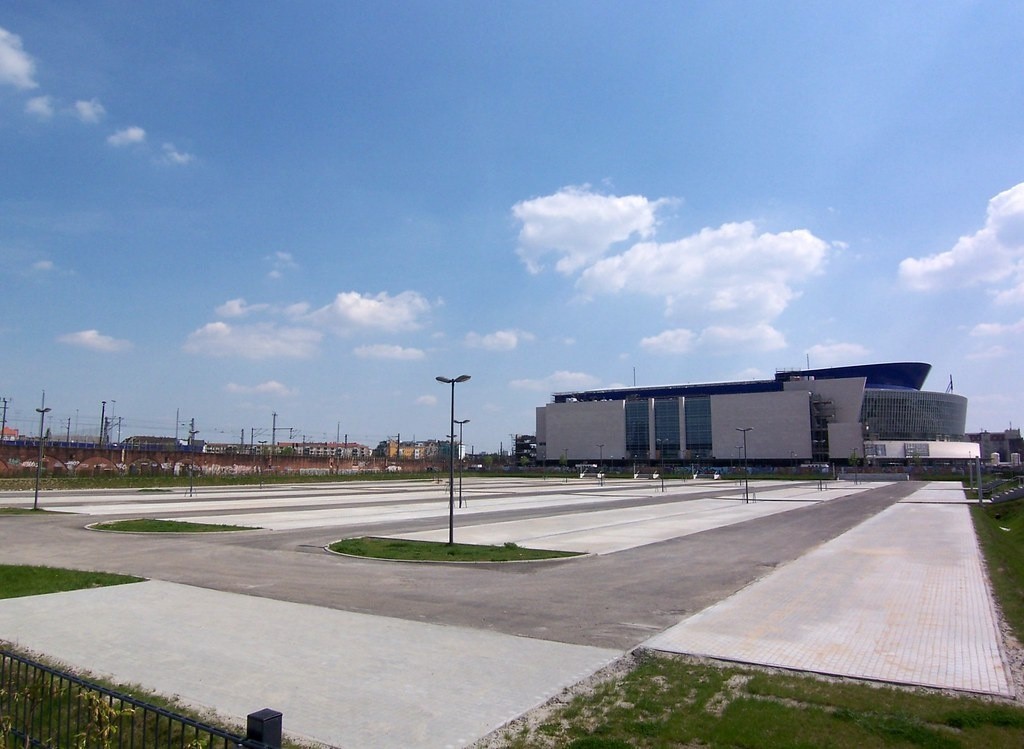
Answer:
[32,407,53,509]
[735,427,754,503]
[435,375,472,546]
[110,400,116,444]
[850,447,858,484]
[258,441,266,488]
[813,439,826,491]
[657,438,669,492]
[188,430,201,497]
[562,448,569,483]
[539,440,547,481]
[734,445,744,486]
[596,444,605,486]
[98,400,107,444]
[454,420,471,509]
